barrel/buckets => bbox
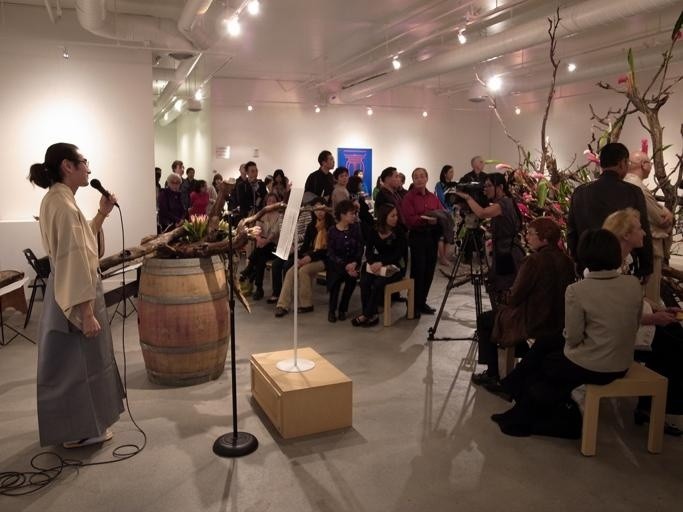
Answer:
[136,254,231,387]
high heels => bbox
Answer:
[634,408,681,438]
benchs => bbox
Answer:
[246,259,415,327]
[527,338,669,456]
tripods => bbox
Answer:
[428,221,491,339]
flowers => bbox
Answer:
[217,218,261,241]
[183,213,210,240]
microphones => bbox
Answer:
[90,179,119,209]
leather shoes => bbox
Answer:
[420,304,436,313]
[328,311,335,322]
[414,311,420,318]
[339,310,346,320]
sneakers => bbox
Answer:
[298,306,314,313]
[361,317,379,327]
[352,314,368,326]
[254,290,264,300]
[483,381,513,403]
[275,307,288,316]
[267,299,278,303]
[472,373,497,385]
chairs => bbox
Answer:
[23,248,46,329]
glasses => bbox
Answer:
[80,160,90,167]
[483,183,494,188]
[170,181,181,184]
[526,231,539,235]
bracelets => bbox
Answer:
[98,208,109,217]
[464,192,470,199]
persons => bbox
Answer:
[273,196,336,317]
[602,207,683,438]
[165,160,185,187]
[471,216,576,386]
[189,179,210,218]
[398,173,407,198]
[373,176,381,217]
[346,176,373,237]
[266,190,312,303]
[240,192,280,300]
[238,162,266,219]
[156,173,187,227]
[325,199,365,322]
[264,175,273,191]
[482,229,644,403]
[305,150,334,206]
[399,168,447,318]
[623,151,673,307]
[155,167,162,199]
[331,167,350,218]
[183,166,197,189]
[433,165,456,266]
[272,169,292,204]
[458,156,493,207]
[207,174,224,215]
[228,164,247,211]
[375,167,416,276]
[354,169,364,178]
[567,142,654,285]
[25,143,127,448]
[447,172,526,310]
[351,203,409,327]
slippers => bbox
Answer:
[63,431,113,448]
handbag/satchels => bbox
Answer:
[366,262,401,278]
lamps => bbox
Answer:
[188,68,202,111]
[458,26,466,44]
[392,55,400,70]
[467,65,488,102]
